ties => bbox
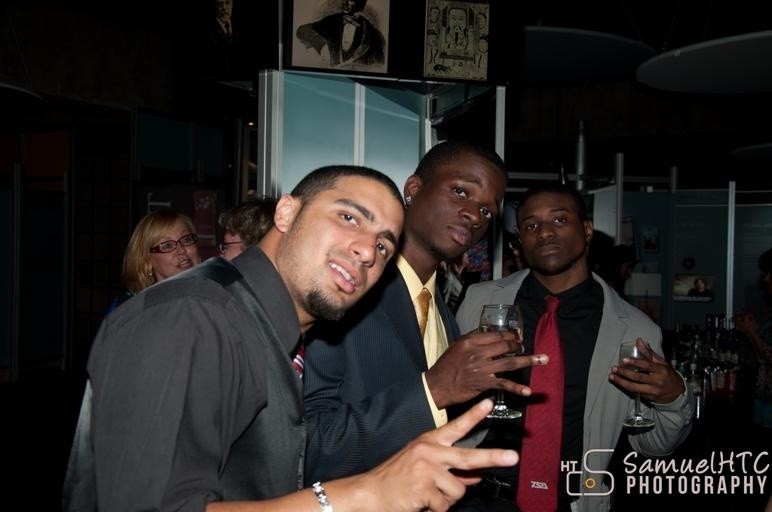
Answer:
[291,340,306,382]
[516,293,566,510]
[415,286,433,340]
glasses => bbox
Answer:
[149,232,199,254]
[219,241,243,253]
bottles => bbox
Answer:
[669,316,742,418]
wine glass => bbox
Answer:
[618,344,654,430]
[479,303,522,419]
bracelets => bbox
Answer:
[312,481,332,512]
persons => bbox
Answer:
[304,137,549,512]
[214,196,278,264]
[688,278,712,298]
[428,3,488,77]
[120,207,203,296]
[204,1,244,81]
[500,225,528,276]
[61,164,520,512]
[296,0,386,70]
